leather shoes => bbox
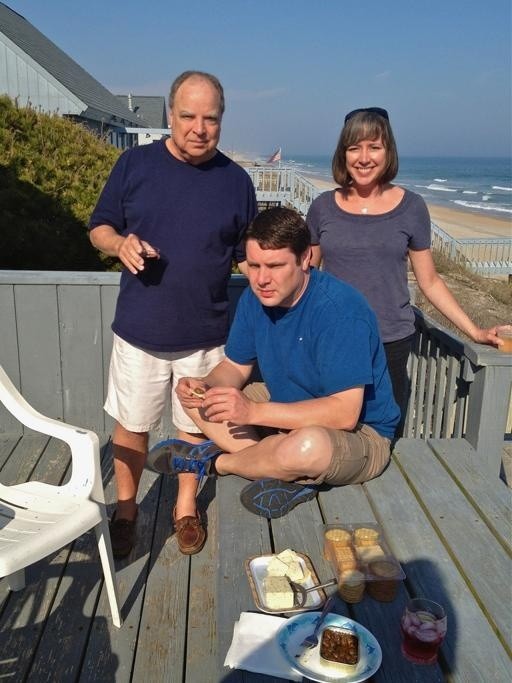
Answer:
[109,506,139,561]
[170,505,207,555]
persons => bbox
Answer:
[145,207,402,520]
[305,111,512,438]
[89,72,260,559]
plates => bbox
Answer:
[272,611,383,682]
[244,550,328,614]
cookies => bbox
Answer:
[192,388,205,398]
[323,528,401,604]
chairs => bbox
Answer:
[0,359,126,630]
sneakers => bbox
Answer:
[146,439,224,477]
[239,478,320,520]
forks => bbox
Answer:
[298,596,336,651]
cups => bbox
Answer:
[495,322,512,353]
[399,597,447,666]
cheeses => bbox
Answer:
[262,549,305,610]
